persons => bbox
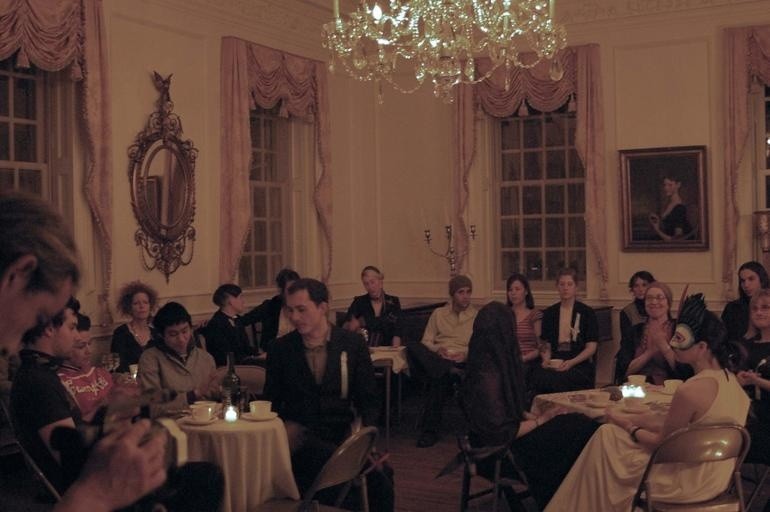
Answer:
[137,302,221,416]
[533,268,600,397]
[263,277,396,511]
[466,302,568,509]
[342,266,405,427]
[720,258,770,465]
[206,284,267,366]
[646,173,696,242]
[8,296,225,512]
[0,189,188,511]
[198,268,300,348]
[57,313,113,416]
[615,282,695,387]
[110,281,157,372]
[506,274,543,387]
[417,275,480,448]
[620,270,655,340]
[546,293,751,510]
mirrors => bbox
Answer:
[128,71,199,284]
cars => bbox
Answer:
[250,401,272,417]
[551,359,564,365]
[129,365,139,382]
[628,375,646,385]
[663,380,683,391]
[624,397,640,408]
[590,392,610,402]
[190,404,219,421]
[194,401,222,416]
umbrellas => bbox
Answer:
[417,431,438,447]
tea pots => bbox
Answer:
[128,71,199,284]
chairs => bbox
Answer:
[1,323,410,512]
[454,370,766,510]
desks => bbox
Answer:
[336,301,447,386]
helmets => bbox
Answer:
[585,401,614,408]
[183,416,219,424]
[621,405,650,413]
[241,412,278,421]
[547,365,564,367]
[190,414,220,416]
[660,390,675,395]
[624,382,650,388]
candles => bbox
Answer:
[442,196,451,227]
[470,196,476,226]
[418,201,429,231]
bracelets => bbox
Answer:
[535,419,539,427]
[631,427,643,443]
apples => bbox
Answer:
[470,196,476,226]
[442,196,451,227]
[418,201,429,231]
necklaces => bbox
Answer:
[371,293,382,303]
[130,323,148,344]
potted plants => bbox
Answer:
[141,176,161,219]
[617,145,711,253]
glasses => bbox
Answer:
[320,0,567,105]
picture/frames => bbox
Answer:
[141,176,161,219]
[336,301,447,386]
[617,145,711,253]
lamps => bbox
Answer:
[320,0,567,105]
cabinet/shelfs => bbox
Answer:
[221,352,240,419]
